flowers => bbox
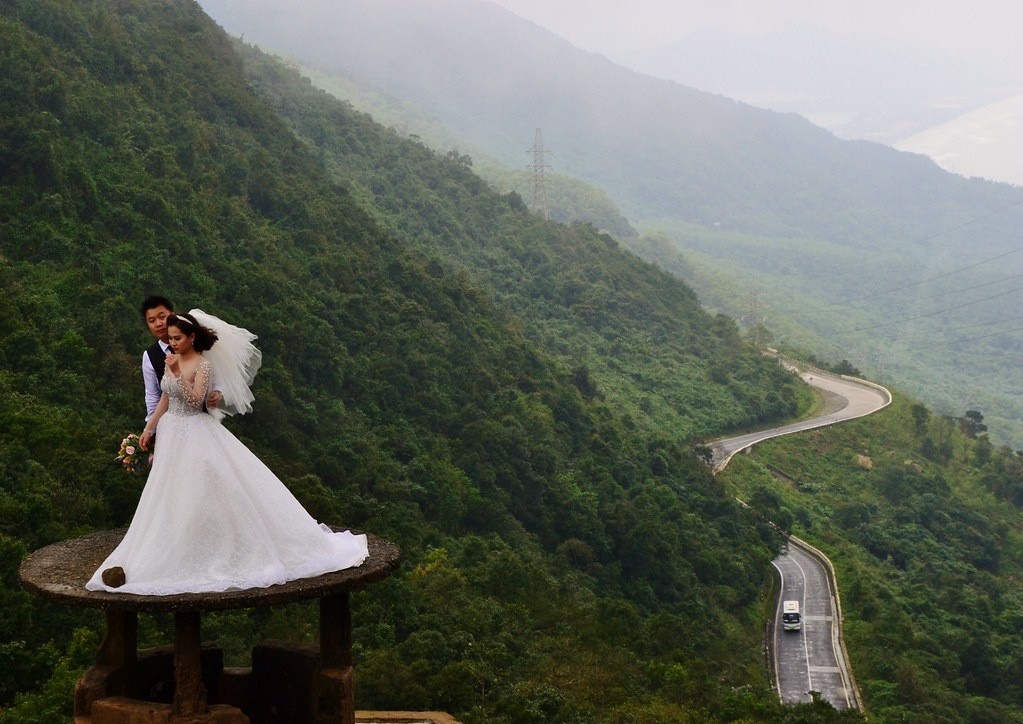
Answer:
[112,433,154,474]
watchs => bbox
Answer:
[218,390,224,401]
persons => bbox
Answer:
[85,294,370,596]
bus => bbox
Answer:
[782,600,801,632]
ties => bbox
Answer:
[167,346,175,354]
